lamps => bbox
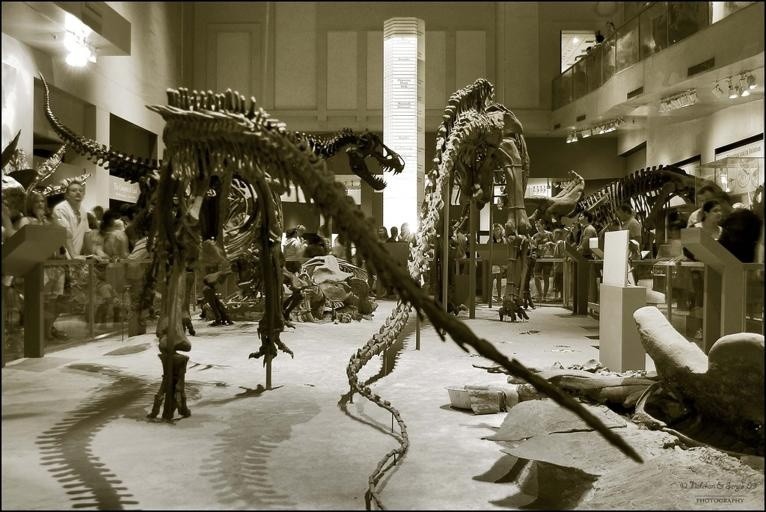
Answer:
[710,68,761,101]
[656,88,700,115]
[566,115,625,145]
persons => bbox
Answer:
[486,199,695,312]
[558,31,617,106]
[685,185,719,230]
[10,191,51,231]
[282,222,420,275]
[86,202,145,331]
[44,180,103,347]
[682,200,724,338]
